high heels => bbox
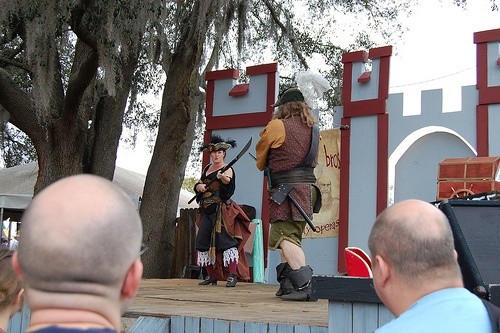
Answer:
[199,275,217,285]
[226,274,237,286]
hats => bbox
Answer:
[270,88,304,108]
[200,134,237,153]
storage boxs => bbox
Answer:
[436,157,500,201]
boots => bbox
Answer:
[280,264,318,301]
[275,262,295,296]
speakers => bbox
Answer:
[437,199,500,309]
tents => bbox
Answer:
[0,162,201,220]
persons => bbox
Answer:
[255,88,319,302]
[368,199,500,333]
[193,135,251,288]
[0,173,145,333]
